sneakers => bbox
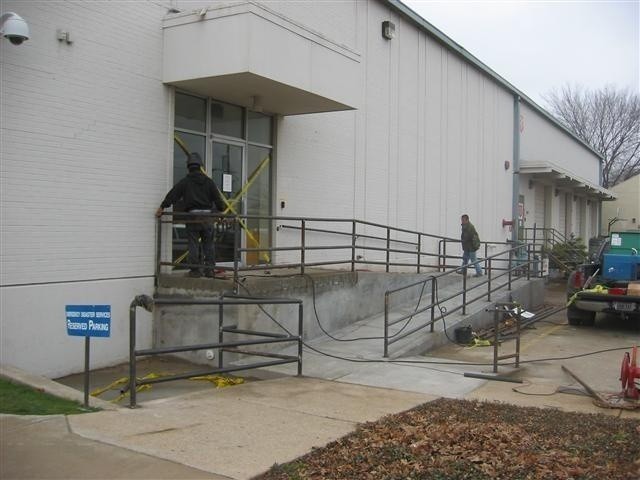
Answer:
[185,271,215,278]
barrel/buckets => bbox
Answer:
[454,326,472,345]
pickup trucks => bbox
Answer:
[566,232,640,326]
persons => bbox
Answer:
[155,152,226,278]
[456,215,484,278]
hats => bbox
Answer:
[188,153,201,165]
